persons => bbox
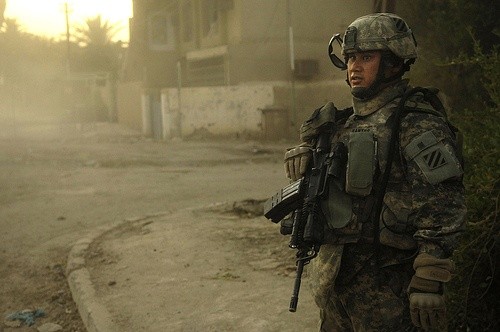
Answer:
[285,13,467,332]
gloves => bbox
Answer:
[284,142,311,181]
[406,254,454,332]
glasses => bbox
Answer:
[327,33,348,71]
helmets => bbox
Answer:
[341,13,418,59]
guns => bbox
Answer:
[263,99,348,313]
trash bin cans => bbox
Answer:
[260,108,288,141]
